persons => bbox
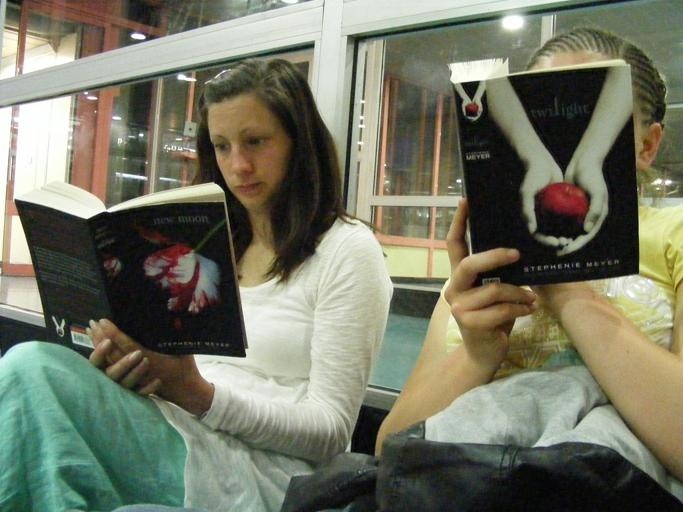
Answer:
[276,25,683,512]
[0,54,395,512]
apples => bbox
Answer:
[465,99,478,116]
[535,183,589,240]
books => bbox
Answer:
[8,180,248,361]
[442,56,643,293]
